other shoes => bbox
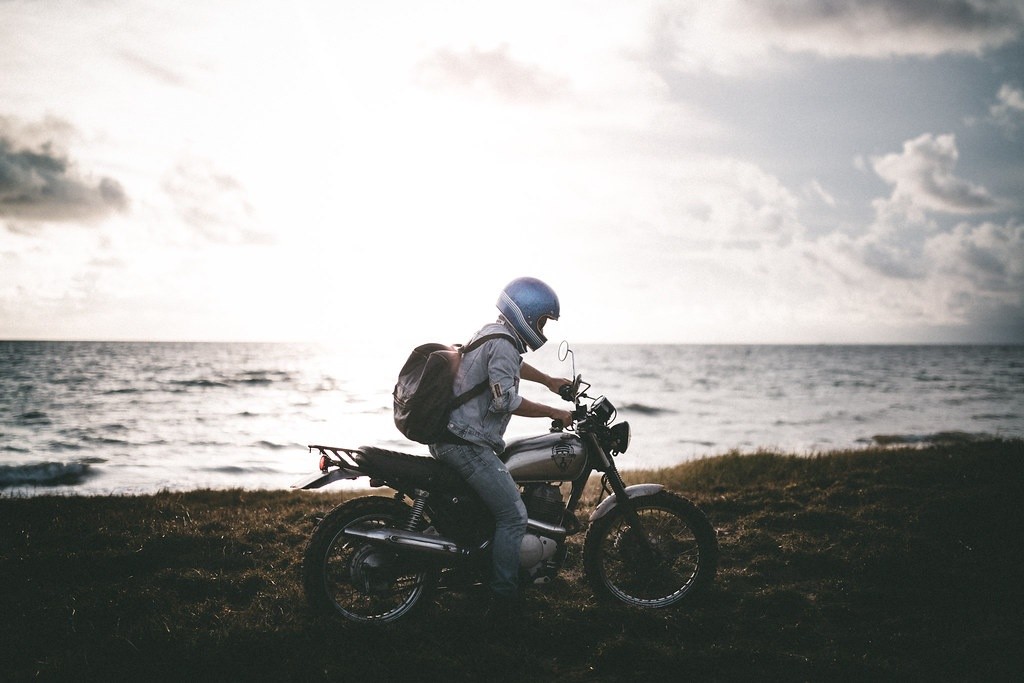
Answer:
[472,601,538,629]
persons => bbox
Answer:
[394,277,573,609]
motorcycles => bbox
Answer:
[298,341,718,640]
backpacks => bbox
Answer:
[394,333,518,446]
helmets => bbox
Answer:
[496,277,559,352]
